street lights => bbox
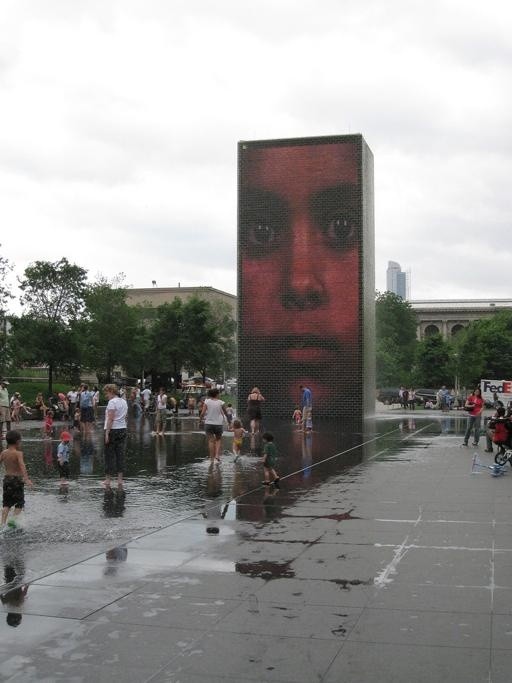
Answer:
[452,352,462,406]
[221,348,227,395]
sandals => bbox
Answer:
[261,480,271,485]
[273,477,279,482]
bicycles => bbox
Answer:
[493,432,511,467]
[471,453,505,477]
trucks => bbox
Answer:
[480,378,512,409]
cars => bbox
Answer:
[97,370,180,392]
[183,376,215,386]
[414,388,455,406]
[376,387,413,406]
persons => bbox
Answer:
[100,384,127,484]
[462,388,512,453]
[102,485,127,579]
[239,144,362,419]
[399,417,490,435]
[197,387,280,485]
[121,384,204,434]
[0,553,32,628]
[1,381,100,433]
[57,431,73,481]
[0,432,32,533]
[264,486,282,524]
[197,495,231,536]
[292,384,313,434]
[398,384,512,415]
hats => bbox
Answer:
[61,432,73,443]
